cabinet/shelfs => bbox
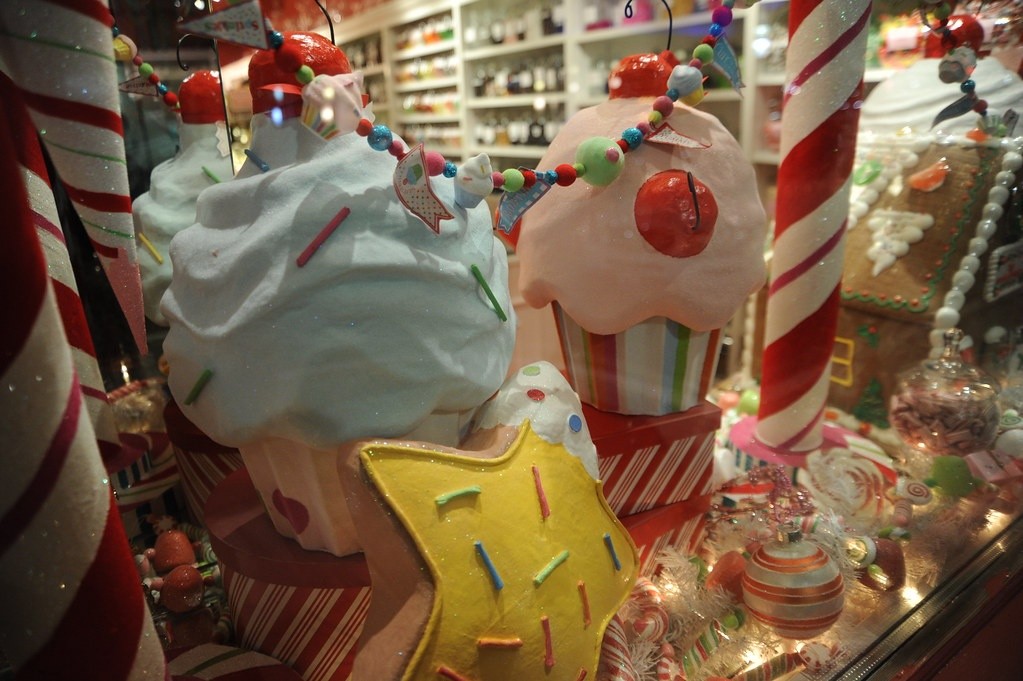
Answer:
[220,0,912,230]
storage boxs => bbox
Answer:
[559,368,723,581]
